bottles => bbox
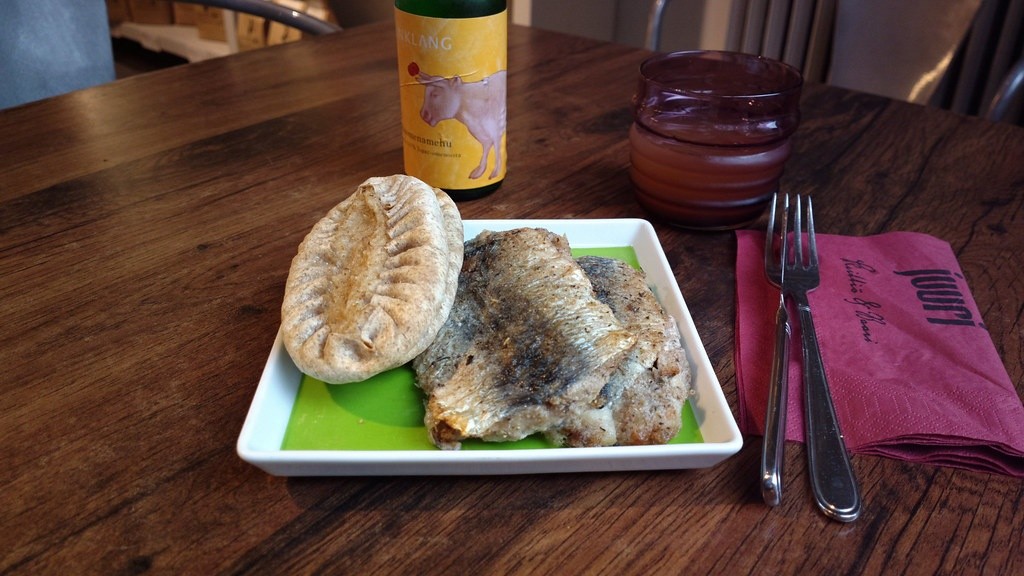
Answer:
[394,0,510,200]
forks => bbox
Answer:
[763,190,864,522]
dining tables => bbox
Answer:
[3,14,1024,575]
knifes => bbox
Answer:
[756,204,791,508]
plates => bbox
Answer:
[231,218,743,476]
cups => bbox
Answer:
[628,50,803,231]
